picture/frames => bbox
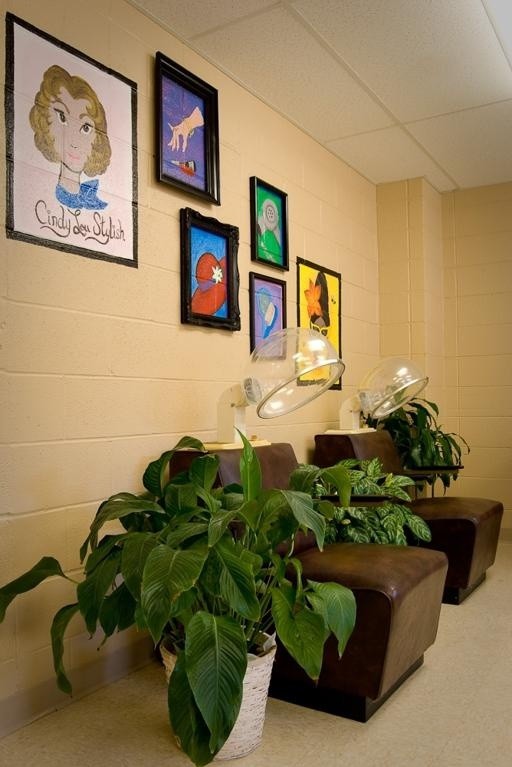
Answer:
[180,207,241,331]
[156,51,221,206]
[249,271,287,360]
[249,175,289,269]
[2,8,137,272]
[295,254,341,390]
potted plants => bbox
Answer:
[0,430,358,767]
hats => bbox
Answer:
[190,250,228,317]
[255,286,279,339]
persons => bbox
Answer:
[166,105,206,154]
[27,62,116,210]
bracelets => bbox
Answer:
[184,118,195,137]
